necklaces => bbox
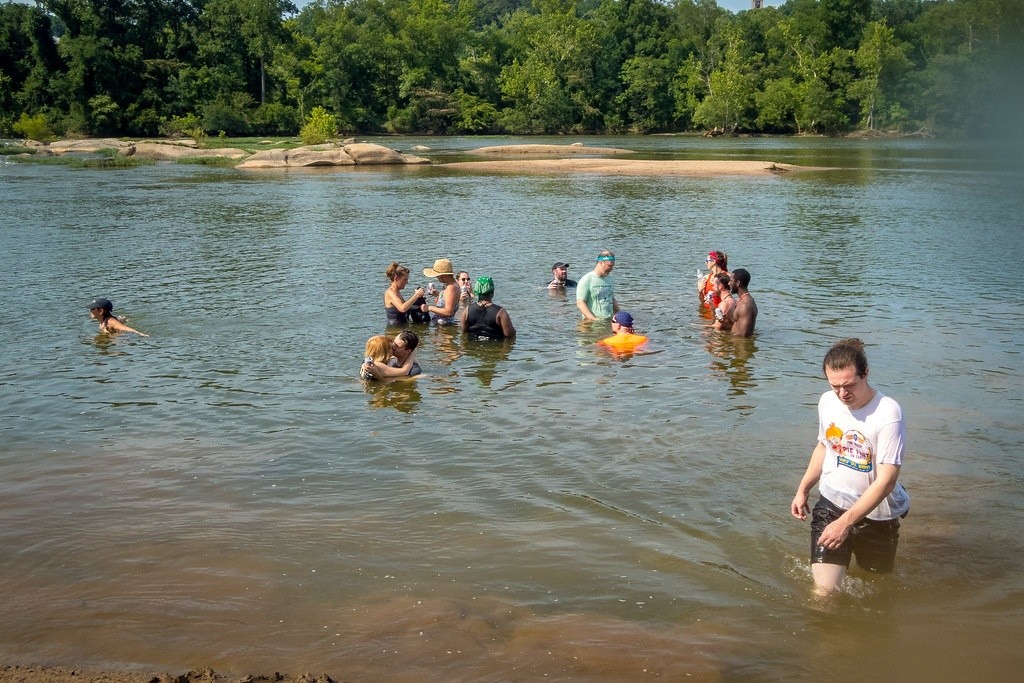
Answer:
[736,293,750,305]
[722,294,731,300]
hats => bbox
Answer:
[614,311,634,326]
[552,262,569,272]
[423,259,455,277]
[85,298,113,312]
[473,276,494,294]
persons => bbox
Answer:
[793,338,911,587]
[698,250,759,337]
[576,251,620,320]
[421,258,462,325]
[462,276,516,338]
[384,262,425,322]
[547,262,578,289]
[603,310,649,345]
[86,298,149,337]
[455,271,474,301]
[359,330,422,380]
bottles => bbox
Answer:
[697,269,703,279]
[364,357,373,380]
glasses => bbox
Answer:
[611,319,618,323]
[462,278,471,282]
[705,258,712,263]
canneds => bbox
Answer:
[461,286,467,292]
[427,283,434,295]
[706,291,714,302]
[366,357,374,366]
